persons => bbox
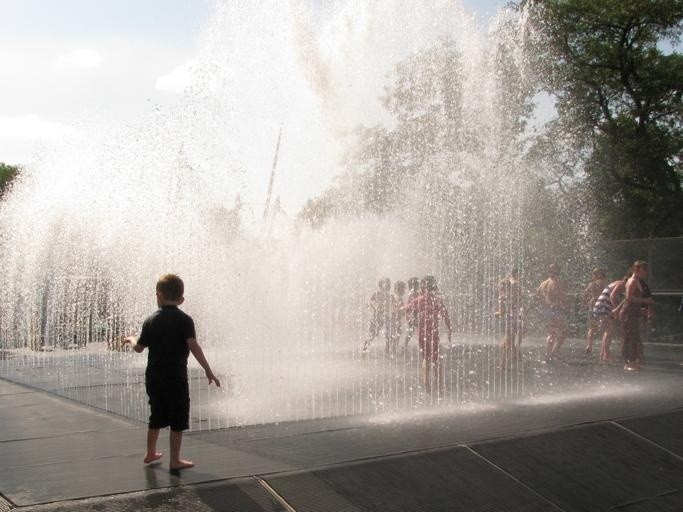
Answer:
[122,274,221,470]
[361,259,656,385]
